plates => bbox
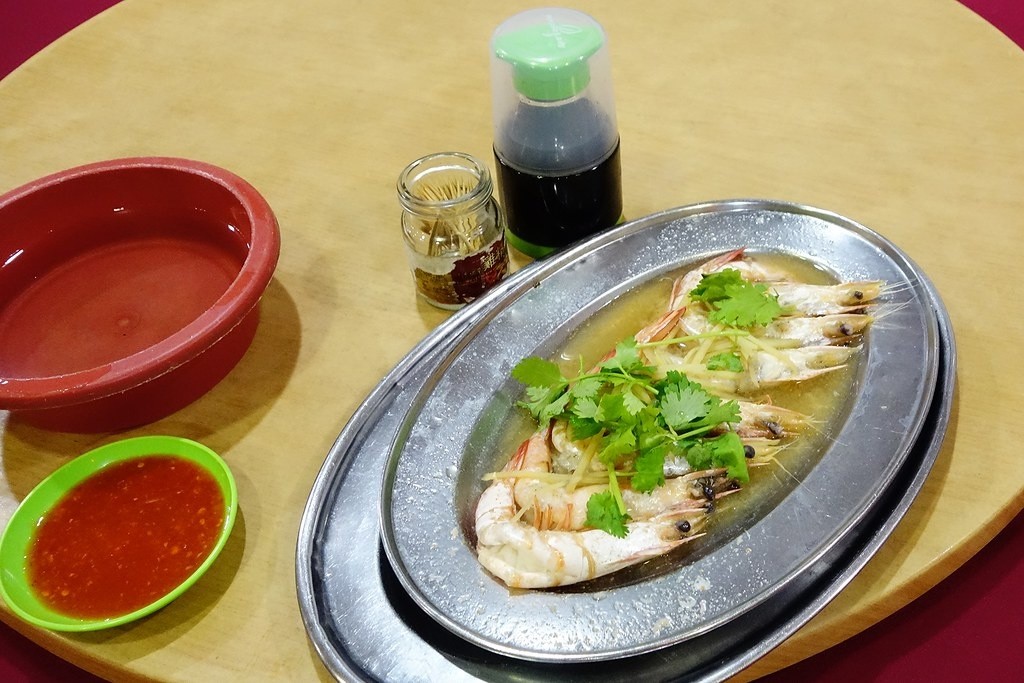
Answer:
[0,433,236,632]
[377,207,941,664]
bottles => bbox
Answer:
[396,152,510,310]
[490,7,621,260]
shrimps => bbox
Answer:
[472,244,917,589]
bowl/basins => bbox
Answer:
[0,156,280,434]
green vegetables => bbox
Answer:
[507,268,805,539]
